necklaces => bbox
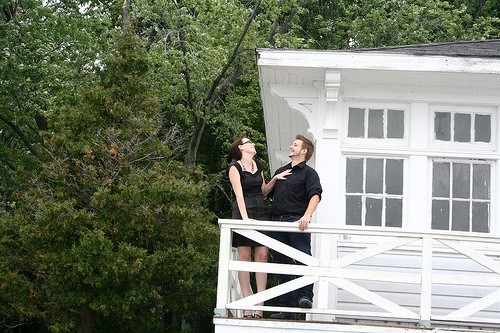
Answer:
[240,159,254,175]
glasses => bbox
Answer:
[241,140,252,145]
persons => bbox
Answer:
[228,137,292,319]
[264,135,322,320]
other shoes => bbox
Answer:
[299,296,312,309]
[254,314,263,319]
[243,314,254,319]
[270,312,295,320]
